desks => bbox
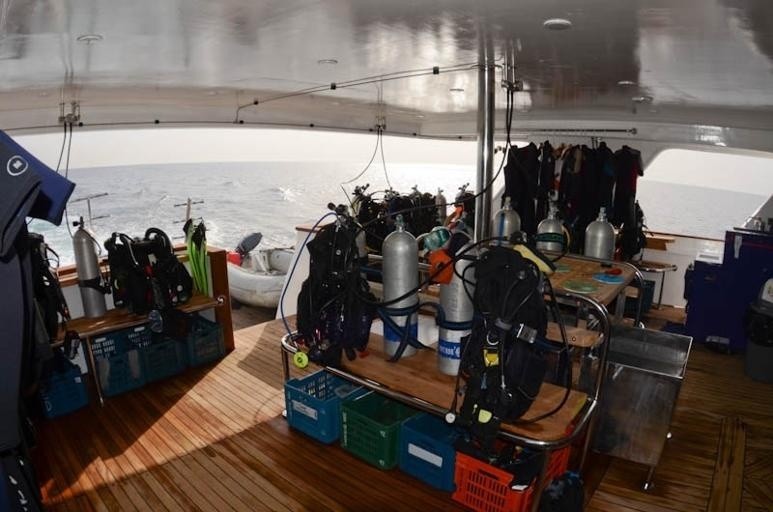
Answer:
[544,256,635,326]
[589,326,694,491]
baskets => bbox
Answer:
[84,314,226,399]
[284,368,572,511]
[35,354,91,420]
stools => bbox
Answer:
[633,260,676,311]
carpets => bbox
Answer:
[706,418,771,512]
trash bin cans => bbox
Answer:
[745,277,773,382]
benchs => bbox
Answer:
[279,253,612,512]
[564,254,644,327]
[51,244,234,406]
